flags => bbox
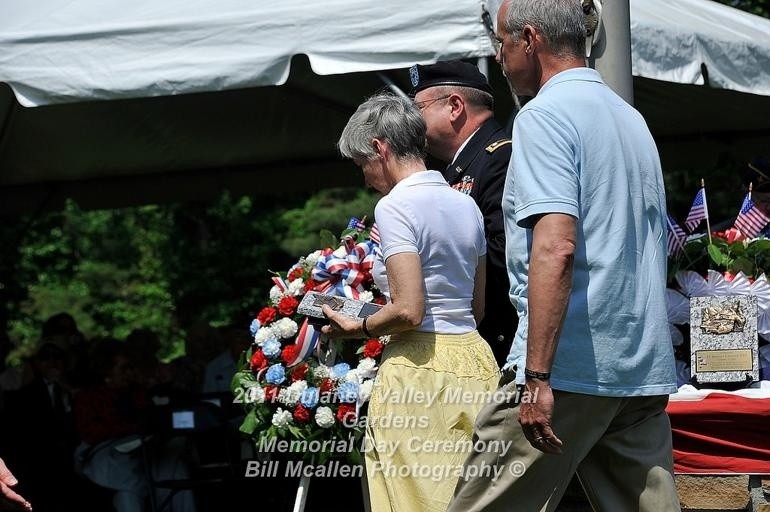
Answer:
[684,189,706,233]
[667,213,686,258]
[734,192,770,239]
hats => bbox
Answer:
[407,60,495,99]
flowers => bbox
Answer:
[233,249,392,467]
[677,232,770,278]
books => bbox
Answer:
[689,295,760,385]
[297,289,384,332]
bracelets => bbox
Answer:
[363,316,372,337]
[525,368,551,380]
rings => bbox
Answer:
[530,425,540,431]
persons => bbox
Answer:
[308,93,503,512]
[407,60,518,368]
[447,0,682,512]
